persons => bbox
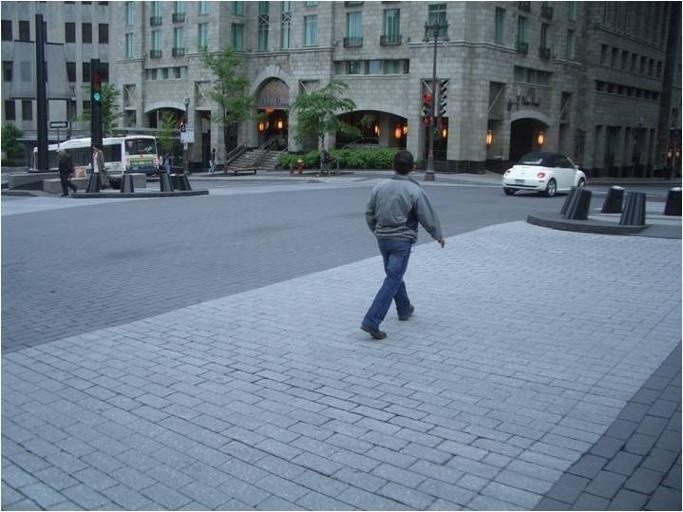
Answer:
[88,142,108,192]
[208,148,217,174]
[360,150,445,340]
[152,152,172,177]
[55,147,78,197]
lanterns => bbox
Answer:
[395,122,415,141]
[373,123,380,138]
[536,131,545,147]
[277,120,283,131]
[258,122,265,132]
[486,131,494,146]
[441,128,447,139]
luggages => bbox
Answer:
[103,169,121,190]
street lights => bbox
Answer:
[668,146,680,179]
[183,95,190,174]
[420,20,449,181]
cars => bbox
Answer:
[502,151,587,197]
[342,137,378,150]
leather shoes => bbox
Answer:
[398,304,414,321]
[360,321,386,340]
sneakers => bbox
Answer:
[60,185,89,198]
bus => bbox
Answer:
[34,135,159,179]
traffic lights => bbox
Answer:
[423,92,434,128]
[90,58,103,105]
[438,79,448,117]
